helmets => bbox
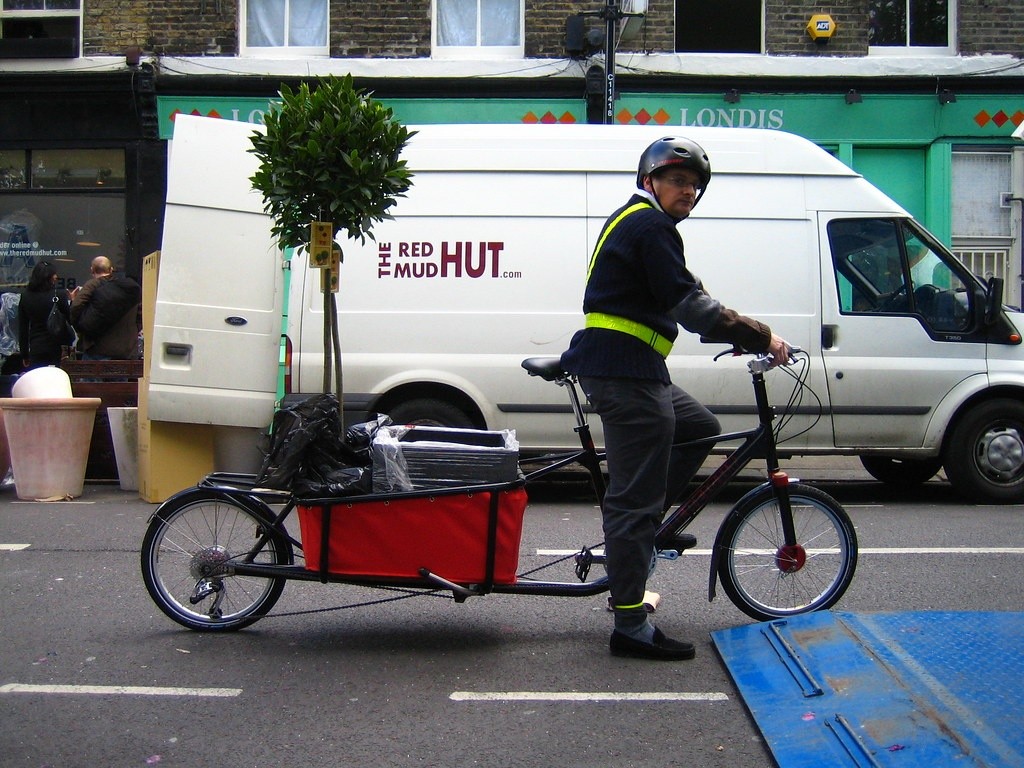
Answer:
[636,135,712,189]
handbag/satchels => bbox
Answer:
[73,273,140,337]
[47,287,76,346]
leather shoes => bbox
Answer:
[609,626,695,661]
[655,523,696,550]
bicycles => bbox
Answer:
[137,333,860,634]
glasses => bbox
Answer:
[41,261,48,272]
[655,172,701,189]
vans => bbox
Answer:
[145,111,1024,509]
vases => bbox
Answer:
[0,398,101,500]
[107,406,141,491]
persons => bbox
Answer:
[17,261,77,369]
[67,256,142,383]
[562,133,795,662]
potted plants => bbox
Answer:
[244,73,419,495]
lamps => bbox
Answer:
[95,169,111,185]
[724,88,741,104]
[846,87,863,105]
[939,88,956,104]
